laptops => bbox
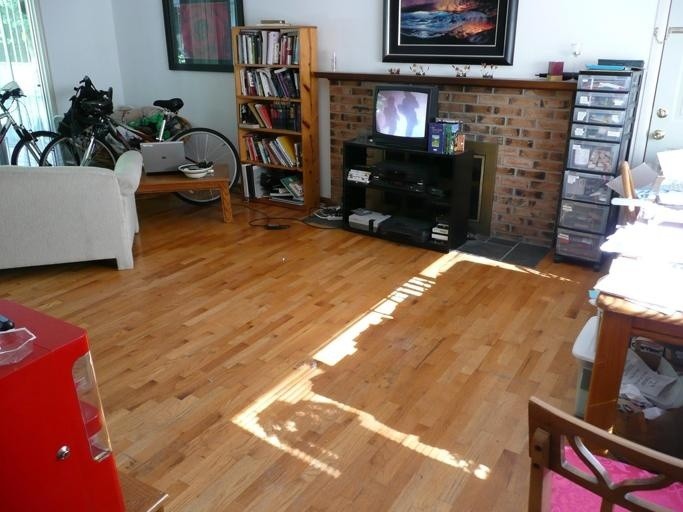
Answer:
[140,141,186,176]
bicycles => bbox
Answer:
[0,75,242,208]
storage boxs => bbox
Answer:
[556,75,639,264]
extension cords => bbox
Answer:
[327,214,343,221]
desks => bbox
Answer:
[583,176,683,458]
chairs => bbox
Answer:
[524,396,683,512]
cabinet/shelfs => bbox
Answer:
[552,71,644,271]
[342,134,474,252]
[229,26,321,213]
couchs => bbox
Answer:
[53,105,193,168]
[1,149,144,271]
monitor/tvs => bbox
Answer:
[372,85,439,151]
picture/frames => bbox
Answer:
[161,0,247,72]
[383,0,520,66]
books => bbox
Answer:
[237,28,303,206]
[260,20,285,24]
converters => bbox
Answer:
[265,223,287,230]
